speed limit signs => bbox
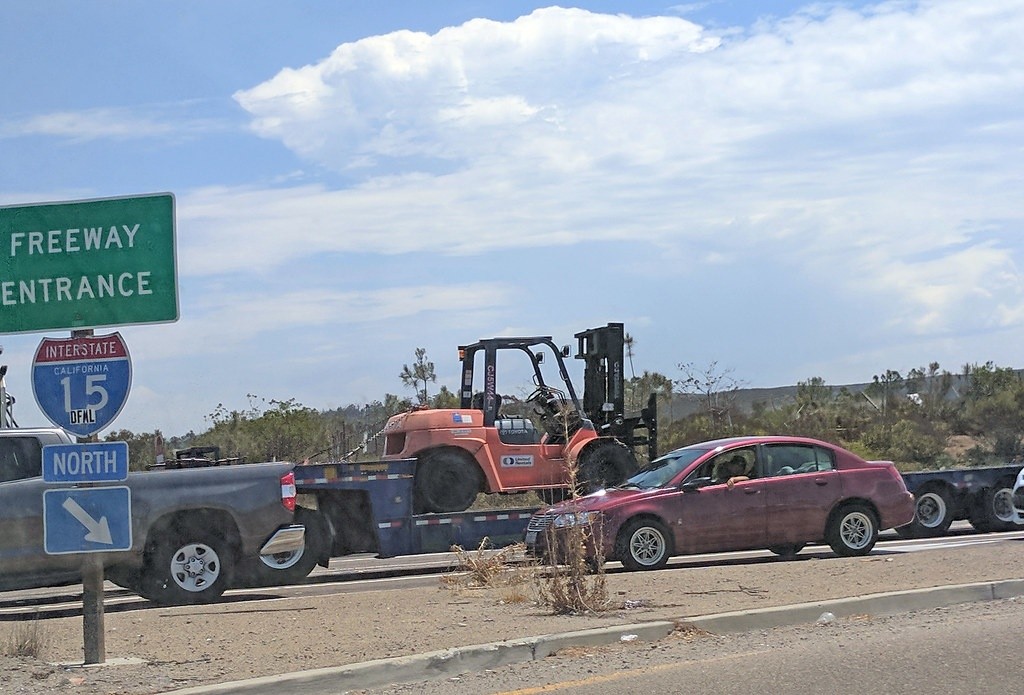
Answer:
[30,332,132,438]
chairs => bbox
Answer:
[714,462,731,484]
[473,392,540,443]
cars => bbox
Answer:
[527,434,915,570]
[1012,467,1024,526]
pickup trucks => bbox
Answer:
[0,427,302,608]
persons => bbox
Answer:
[716,455,750,488]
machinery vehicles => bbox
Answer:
[140,443,1024,587]
[383,321,660,503]
